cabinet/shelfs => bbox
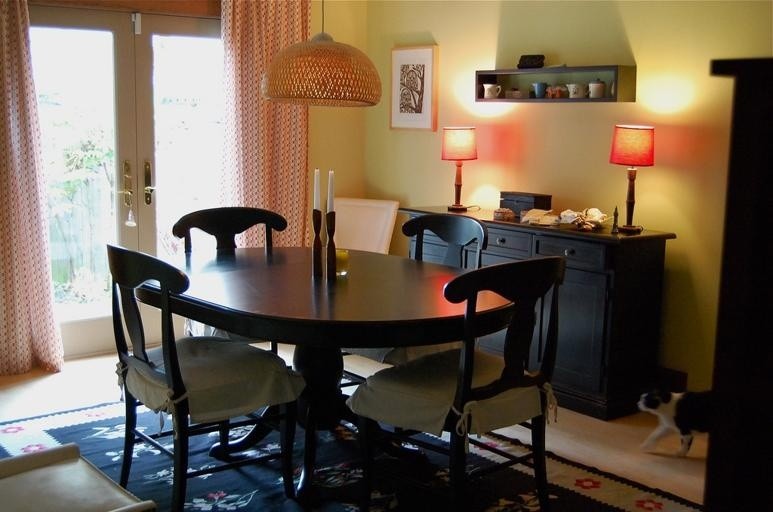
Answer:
[409,207,677,420]
[475,64,636,103]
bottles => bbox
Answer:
[587,77,605,98]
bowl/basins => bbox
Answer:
[504,89,520,98]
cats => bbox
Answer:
[635,385,713,459]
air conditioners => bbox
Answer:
[261,0,382,105]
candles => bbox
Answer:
[326,169,334,212]
[314,168,320,210]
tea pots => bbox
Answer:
[565,83,590,99]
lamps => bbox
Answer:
[441,126,478,211]
[609,124,655,232]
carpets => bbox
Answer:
[0,366,703,512]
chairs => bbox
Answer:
[345,256,566,511]
[172,207,287,354]
[382,214,488,364]
[108,244,306,512]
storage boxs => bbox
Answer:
[499,191,552,215]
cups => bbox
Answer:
[334,248,349,277]
[481,82,501,98]
[529,82,549,98]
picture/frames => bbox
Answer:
[389,45,439,131]
